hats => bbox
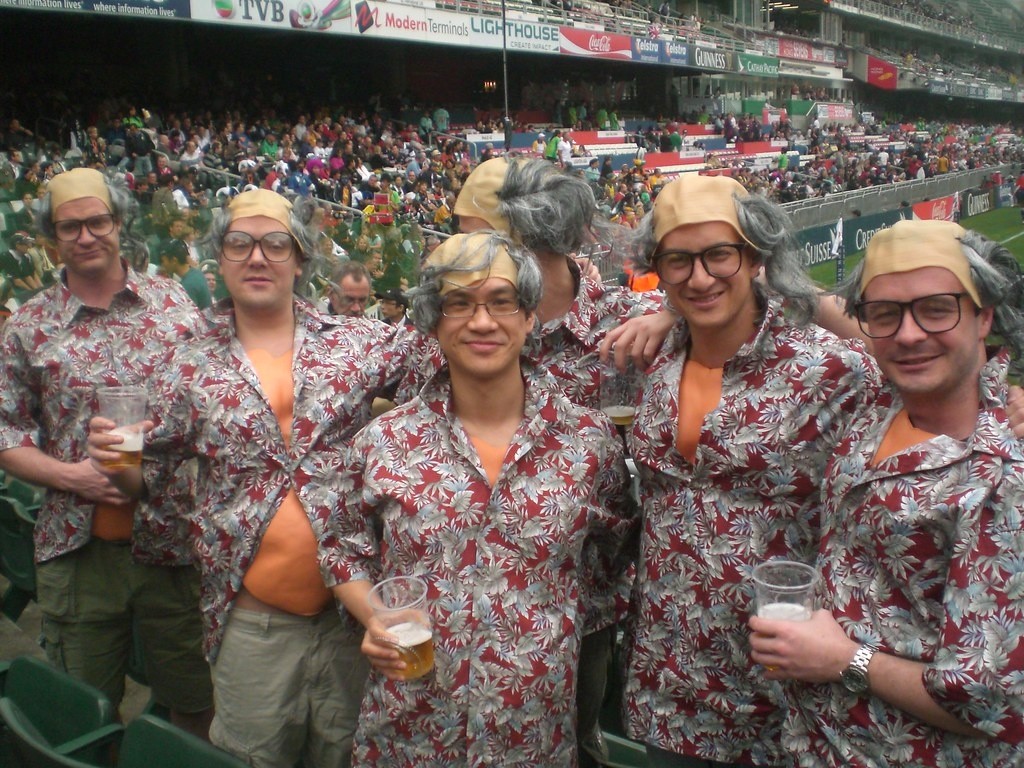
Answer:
[429,162,439,166]
[10,231,35,245]
[265,129,280,135]
[374,287,408,307]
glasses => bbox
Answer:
[653,242,749,285]
[442,295,522,317]
[53,213,114,241]
[855,292,969,338]
[379,301,397,306]
[222,231,295,263]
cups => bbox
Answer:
[367,575,434,681]
[595,338,644,426]
[95,384,148,472]
[751,560,820,673]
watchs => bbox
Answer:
[839,643,879,693]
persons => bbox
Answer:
[320,148,682,767]
[624,173,1024,768]
[0,0,1024,326]
[0,167,214,743]
[87,188,423,768]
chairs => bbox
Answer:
[0,471,257,768]
